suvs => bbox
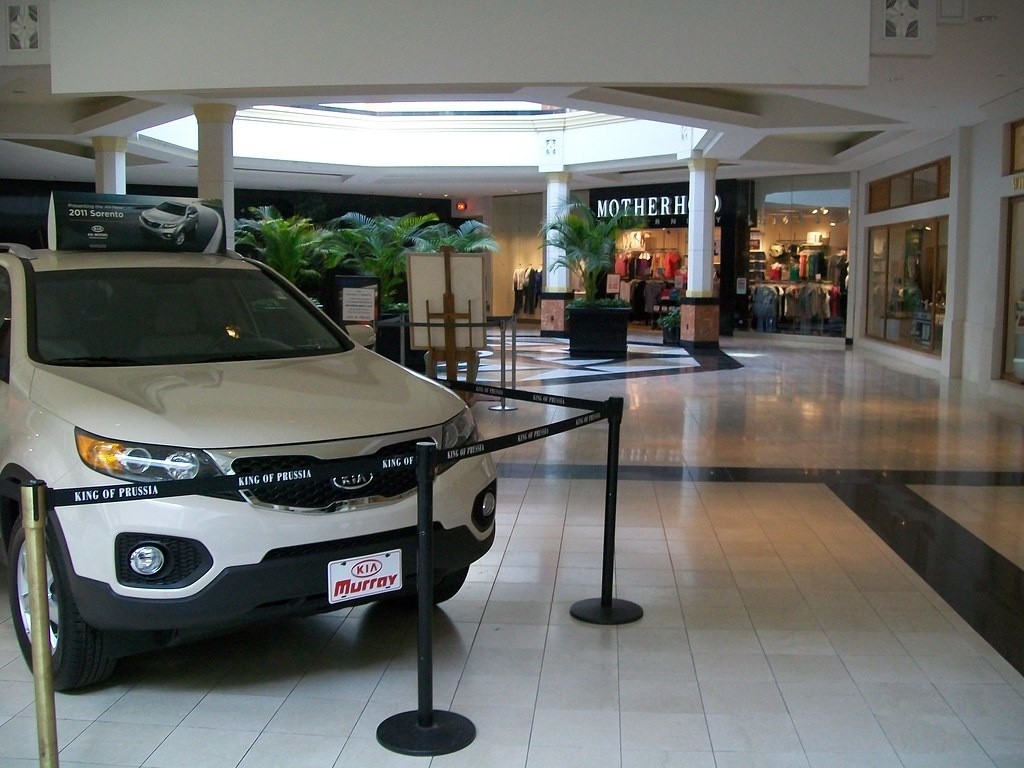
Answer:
[0,242,496,690]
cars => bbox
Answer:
[137,200,200,245]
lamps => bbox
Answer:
[820,207,828,214]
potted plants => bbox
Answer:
[233,206,501,371]
[537,196,648,359]
[657,307,680,345]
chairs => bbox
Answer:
[32,304,88,361]
[140,300,226,358]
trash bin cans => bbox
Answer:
[378,314,428,374]
